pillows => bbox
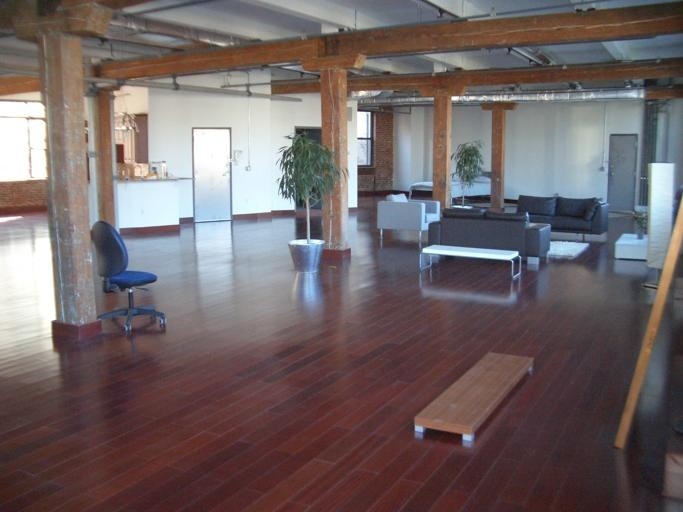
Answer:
[453,174,491,183]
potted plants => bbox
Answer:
[275,131,349,274]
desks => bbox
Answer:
[614,233,647,261]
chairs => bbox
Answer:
[377,194,441,249]
[89,221,166,335]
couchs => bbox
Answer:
[428,208,551,264]
[502,195,610,243]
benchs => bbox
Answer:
[419,245,522,280]
[419,266,522,306]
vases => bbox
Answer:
[636,223,644,239]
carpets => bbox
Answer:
[548,241,590,259]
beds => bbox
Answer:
[408,171,492,200]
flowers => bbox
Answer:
[631,212,647,234]
